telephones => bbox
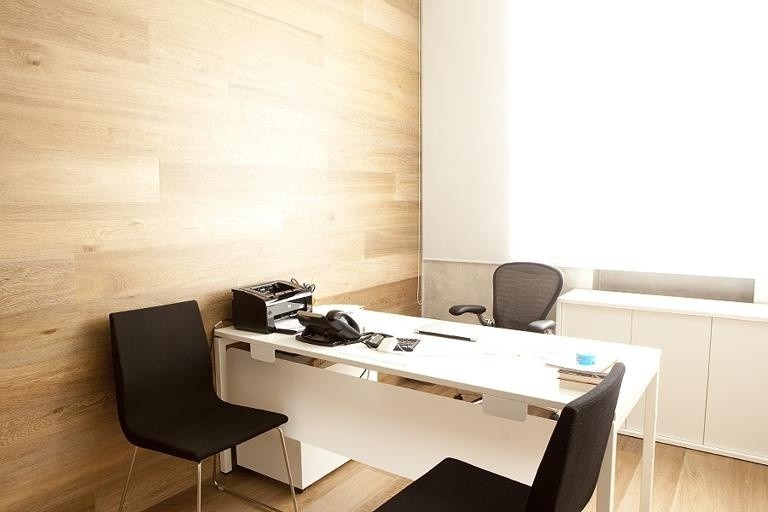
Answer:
[296,309,361,340]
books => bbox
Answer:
[545,353,620,385]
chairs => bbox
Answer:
[107,302,300,510]
[371,358,628,512]
[448,261,565,419]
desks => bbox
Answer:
[210,303,662,512]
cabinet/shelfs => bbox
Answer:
[555,287,768,465]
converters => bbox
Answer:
[377,337,398,352]
[366,334,385,348]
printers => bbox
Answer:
[232,280,312,335]
